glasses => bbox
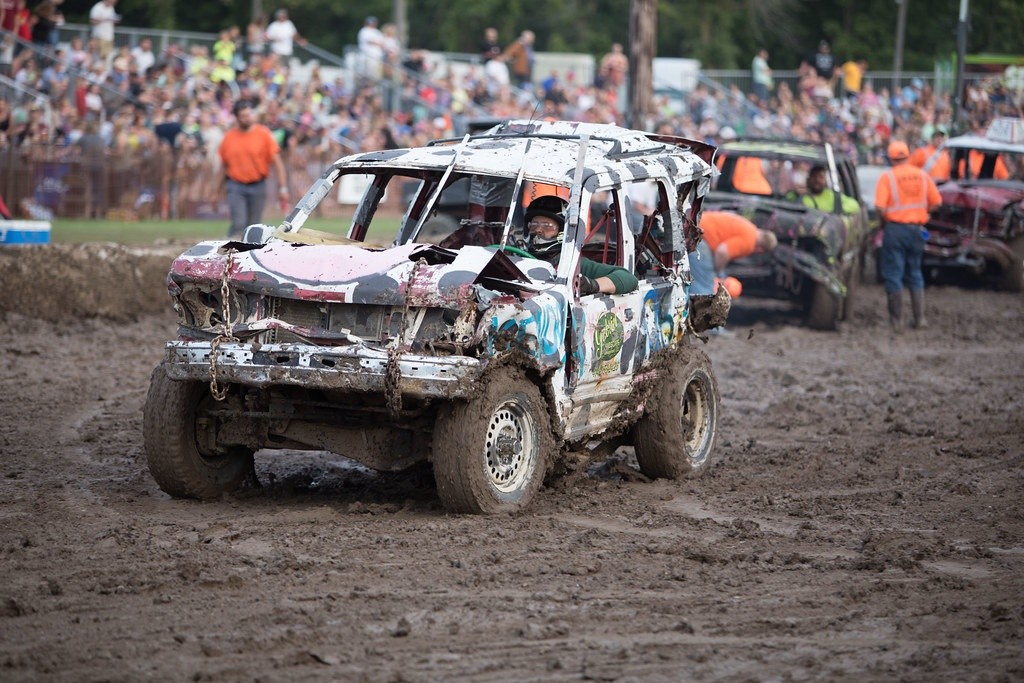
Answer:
[527,222,560,231]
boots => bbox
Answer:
[887,294,904,334]
[911,289,928,328]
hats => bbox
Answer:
[887,141,909,159]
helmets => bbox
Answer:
[522,195,579,268]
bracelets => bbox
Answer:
[278,187,288,192]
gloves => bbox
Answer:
[576,274,600,297]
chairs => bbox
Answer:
[436,224,495,250]
[581,243,617,268]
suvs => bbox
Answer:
[141,119,731,517]
[866,117,1024,290]
[692,139,870,327]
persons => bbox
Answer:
[781,166,859,216]
[908,130,951,187]
[209,98,290,238]
[512,195,639,294]
[958,149,1009,180]
[0,0,1024,220]
[688,210,777,339]
[874,141,943,334]
